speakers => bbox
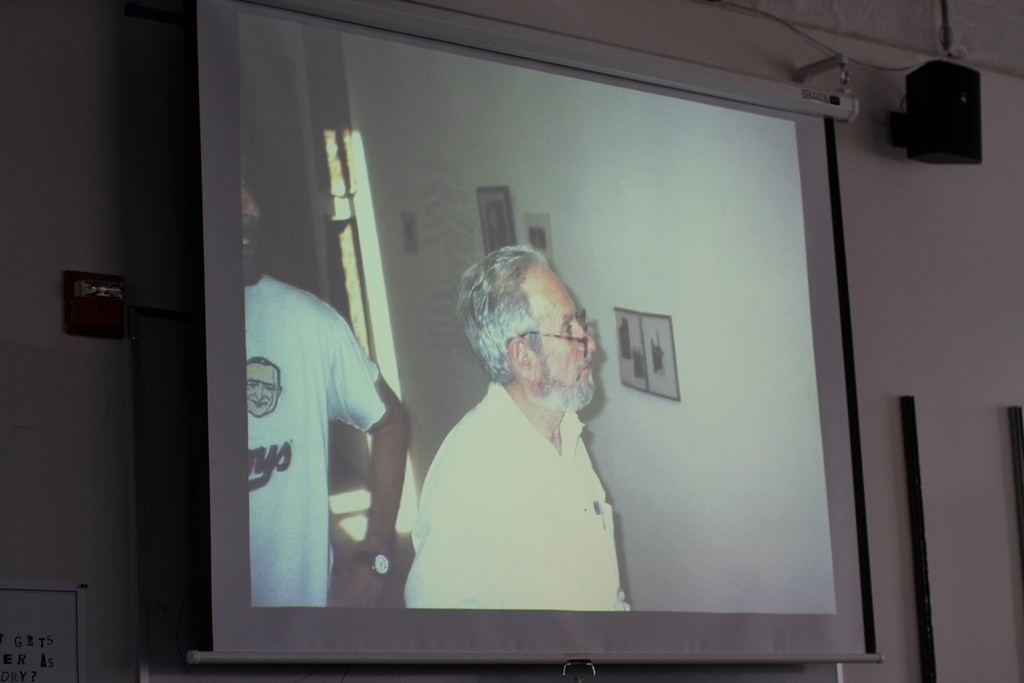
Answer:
[906,58,983,165]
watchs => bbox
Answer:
[361,550,393,577]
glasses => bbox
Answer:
[506,309,589,358]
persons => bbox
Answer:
[237,155,411,609]
[404,244,633,612]
